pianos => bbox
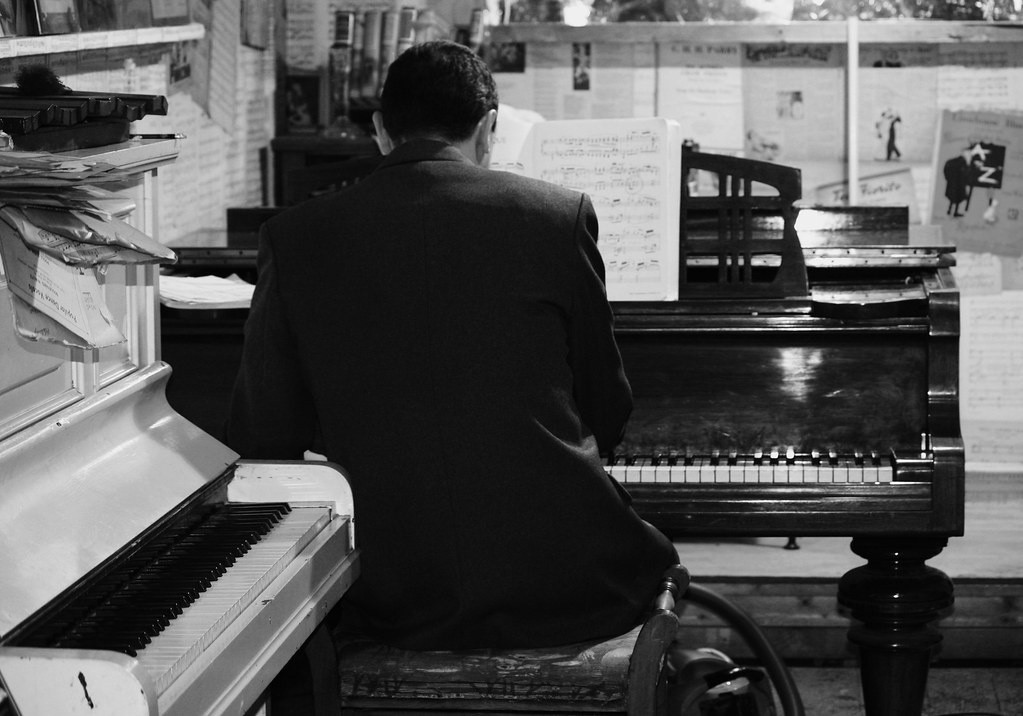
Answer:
[0,361,359,716]
[152,138,967,716]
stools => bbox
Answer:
[309,564,691,716]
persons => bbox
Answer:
[241,35,739,709]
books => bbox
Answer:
[488,102,683,304]
